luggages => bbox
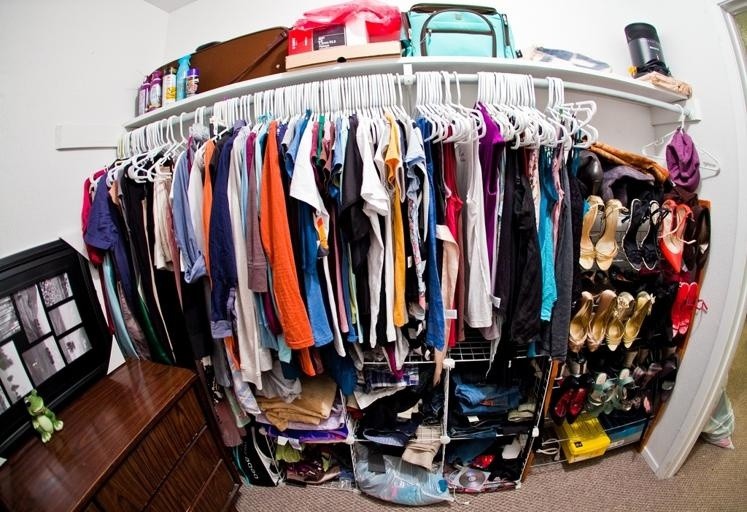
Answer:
[144,25,289,94]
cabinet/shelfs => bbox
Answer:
[1,358,243,512]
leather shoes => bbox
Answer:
[582,273,595,290]
[648,363,676,389]
[640,191,652,197]
[610,269,638,291]
[596,273,615,290]
[685,217,697,270]
[695,205,711,269]
[615,183,628,207]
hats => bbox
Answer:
[666,128,700,192]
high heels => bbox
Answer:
[580,159,603,194]
[622,350,636,367]
[565,374,594,419]
[658,200,683,274]
[568,292,594,354]
[571,359,588,377]
[675,204,691,239]
[639,350,649,366]
[549,376,581,421]
[587,290,617,352]
[663,346,676,359]
[598,359,605,366]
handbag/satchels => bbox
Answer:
[404,1,512,60]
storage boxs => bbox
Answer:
[555,415,611,464]
[597,412,648,451]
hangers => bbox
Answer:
[88,70,605,184]
[639,104,723,177]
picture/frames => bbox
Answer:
[0,236,113,459]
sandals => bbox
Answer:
[679,282,698,335]
[604,376,619,415]
[580,196,604,270]
[606,292,635,352]
[585,371,606,418]
[612,388,631,412]
[621,199,651,272]
[619,367,635,412]
[642,200,669,272]
[623,292,651,349]
[632,367,644,411]
[670,282,689,338]
[595,199,623,271]
